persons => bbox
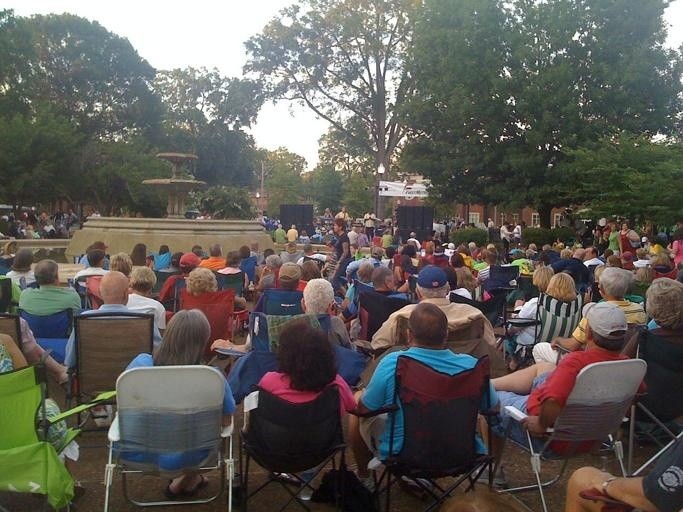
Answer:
[619,278,682,432]
[1,332,85,504]
[65,271,163,428]
[121,309,236,498]
[258,318,355,478]
[470,303,646,487]
[1,201,683,369]
[342,304,503,491]
[565,435,682,511]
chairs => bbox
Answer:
[610,325,682,477]
[238,388,346,510]
[346,355,494,511]
[479,357,646,512]
[0,362,76,512]
[103,364,234,510]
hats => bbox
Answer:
[11,248,34,272]
[372,246,385,259]
[582,302,629,341]
[417,265,448,289]
[179,252,202,267]
[410,231,417,238]
[278,262,301,283]
[641,237,649,243]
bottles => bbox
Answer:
[300,460,315,502]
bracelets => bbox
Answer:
[599,476,621,493]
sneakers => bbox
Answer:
[471,464,512,489]
[347,462,374,492]
[399,476,434,500]
[91,410,112,428]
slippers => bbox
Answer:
[163,474,208,499]
